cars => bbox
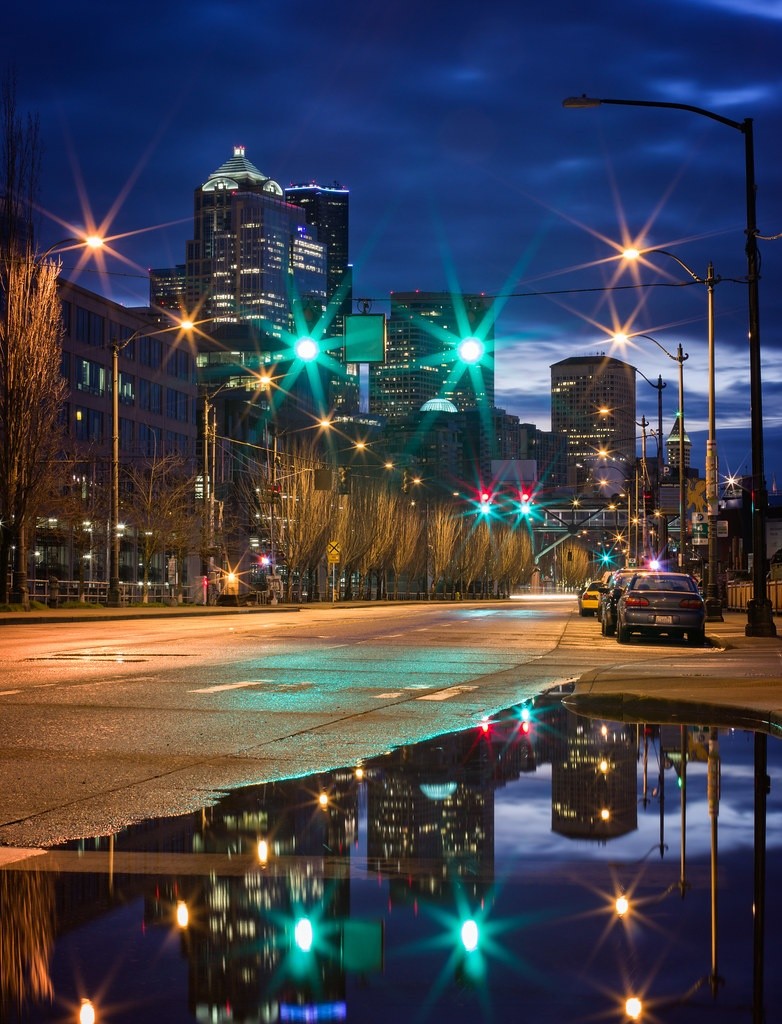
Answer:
[577,569,704,643]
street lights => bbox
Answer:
[111,320,196,604]
[202,374,271,605]
[273,420,464,606]
[599,724,772,1024]
[561,94,777,639]
[10,238,104,610]
